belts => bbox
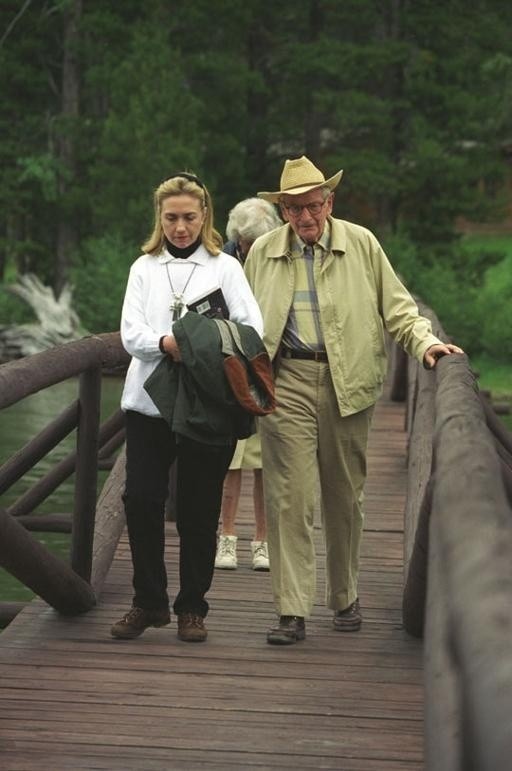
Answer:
[281,349,327,361]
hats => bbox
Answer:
[257,156,343,202]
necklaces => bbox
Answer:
[163,262,197,308]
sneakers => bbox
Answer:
[268,615,305,643]
[250,541,270,570]
[111,604,170,638]
[214,535,238,569]
[333,600,362,631]
[177,612,207,641]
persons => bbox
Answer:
[212,197,286,572]
[111,165,265,640]
[242,154,466,647]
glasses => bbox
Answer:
[281,200,326,216]
